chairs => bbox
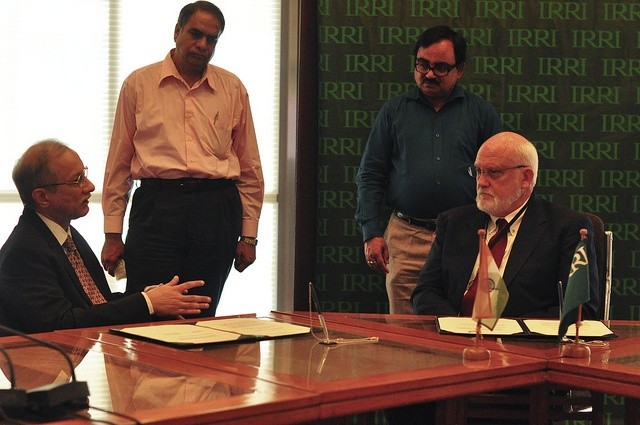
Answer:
[583,210,617,316]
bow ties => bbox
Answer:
[460,220,510,318]
[63,235,107,305]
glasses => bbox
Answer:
[36,166,89,189]
[467,165,531,176]
[415,63,456,77]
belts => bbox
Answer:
[396,211,437,231]
[140,181,234,192]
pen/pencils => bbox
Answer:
[308,282,331,341]
[558,279,568,344]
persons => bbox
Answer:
[0,140,212,332]
[411,131,600,321]
[100,0,263,317]
[355,26,506,315]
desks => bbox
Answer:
[0,311,635,425]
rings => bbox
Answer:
[369,260,375,264]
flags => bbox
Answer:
[472,234,509,331]
[557,235,589,344]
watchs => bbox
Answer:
[241,237,258,247]
